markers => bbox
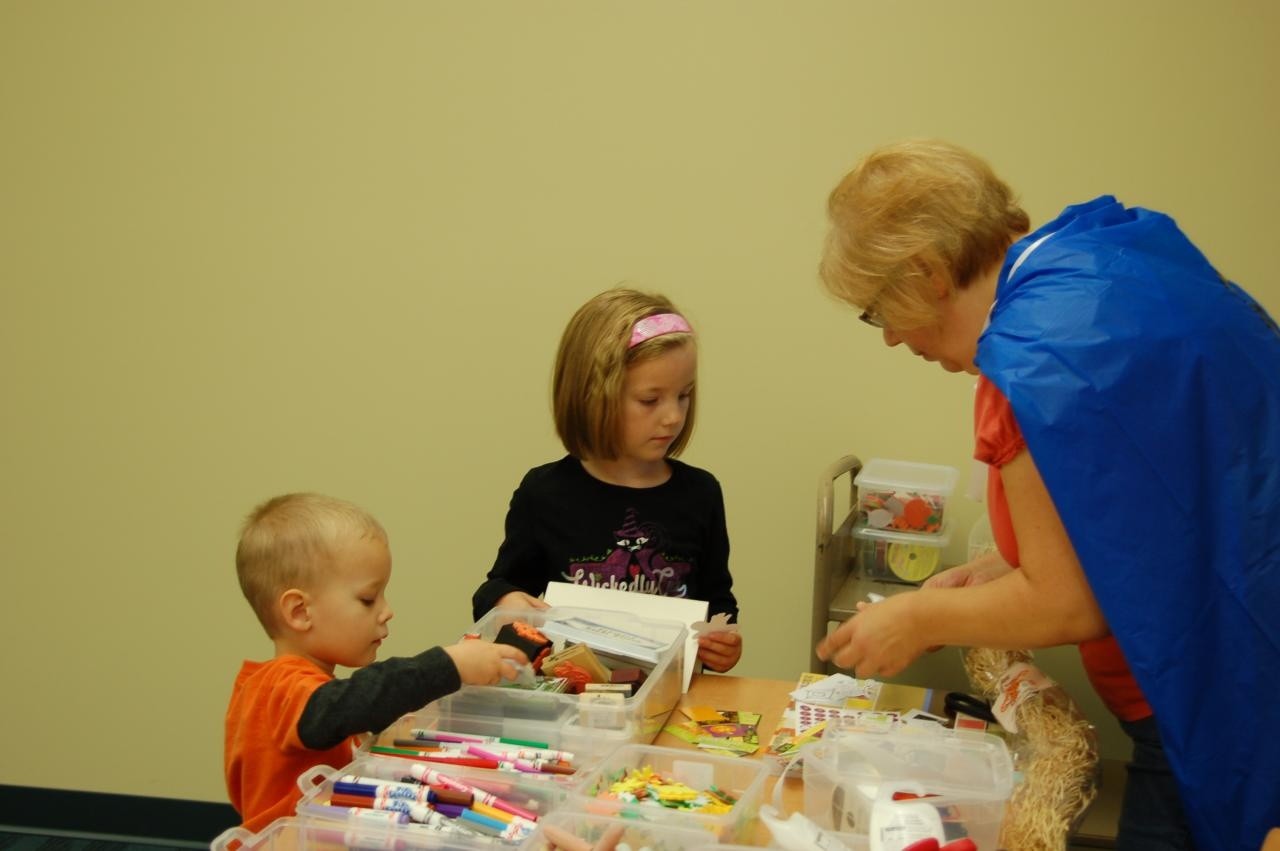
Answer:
[309,729,576,851]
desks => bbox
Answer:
[636,673,989,849]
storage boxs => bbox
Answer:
[211,604,1015,850]
[852,459,957,585]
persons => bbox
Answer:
[470,289,744,673]
[814,140,1280,851]
[224,492,529,851]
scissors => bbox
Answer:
[944,690,999,725]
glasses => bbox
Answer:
[858,264,895,329]
